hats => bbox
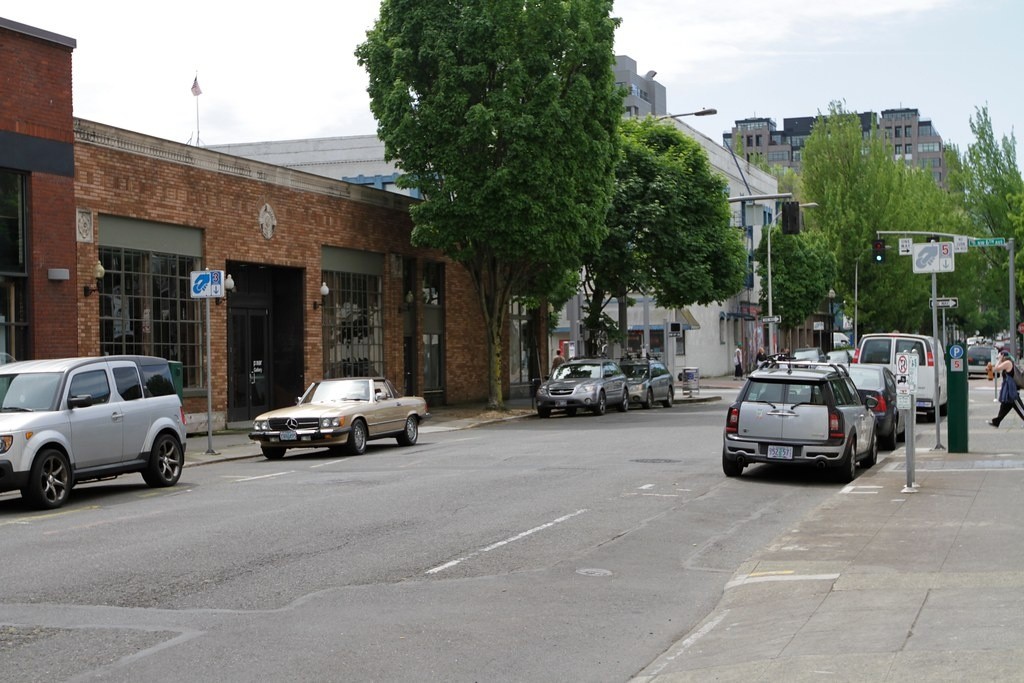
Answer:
[999,350,1009,356]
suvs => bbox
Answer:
[1,354,187,512]
[721,356,880,484]
[614,357,675,409]
[967,346,1000,378]
[535,358,631,417]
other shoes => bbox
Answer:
[986,420,999,429]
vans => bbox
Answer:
[852,333,948,422]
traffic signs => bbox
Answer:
[762,315,781,323]
[929,297,958,309]
[898,238,913,255]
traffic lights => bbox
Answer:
[872,239,886,264]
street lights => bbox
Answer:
[641,108,718,357]
[854,243,893,348]
[768,202,820,356]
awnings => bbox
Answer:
[549,298,701,332]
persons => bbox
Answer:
[755,347,767,367]
[734,343,745,381]
[550,349,566,376]
[986,350,1024,428]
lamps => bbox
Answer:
[314,281,330,309]
[215,274,234,305]
[398,291,414,313]
[84,260,105,296]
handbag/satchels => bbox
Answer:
[1014,364,1024,389]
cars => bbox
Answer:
[826,349,852,363]
[247,375,430,462]
[790,347,826,369]
[807,361,905,451]
